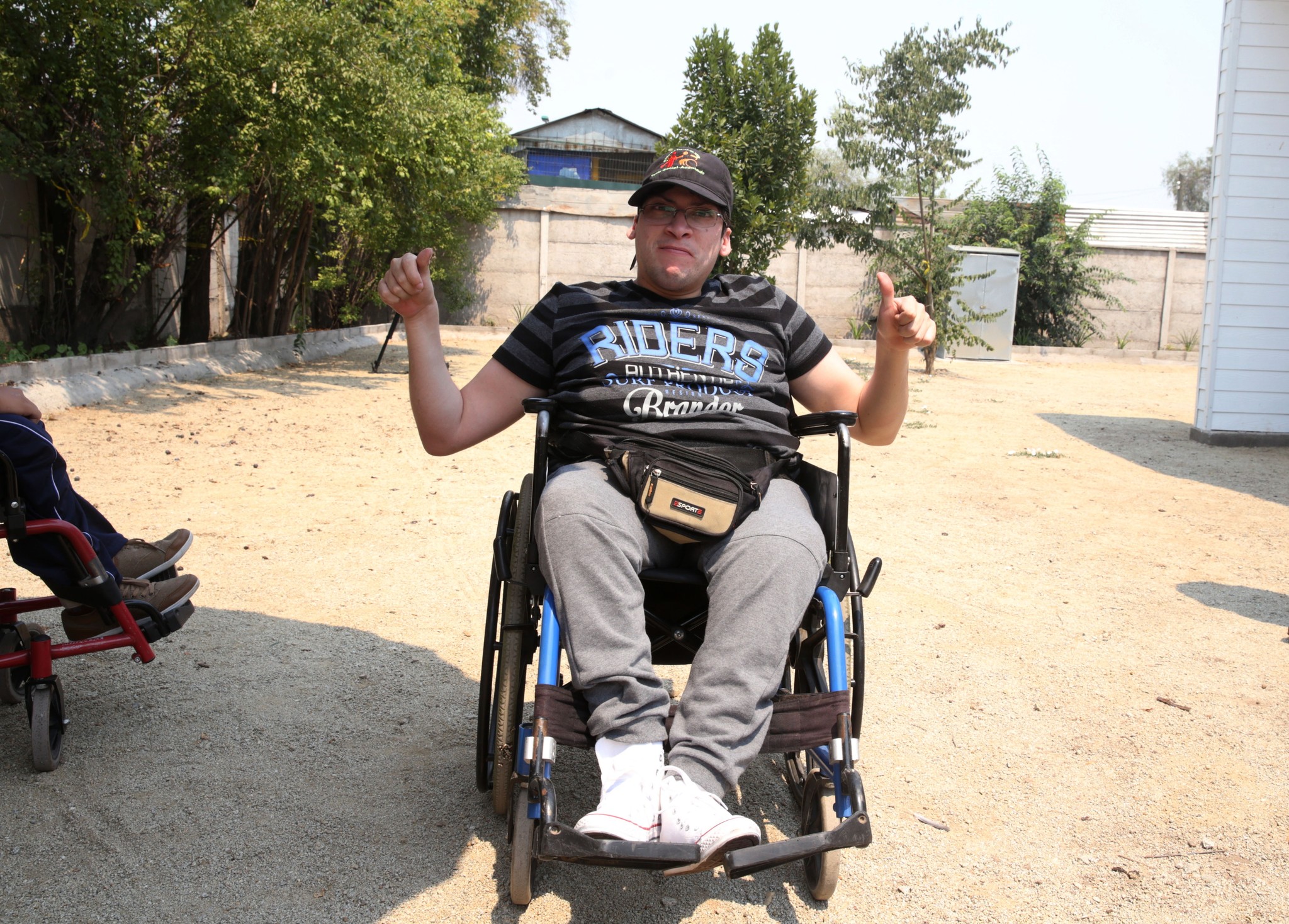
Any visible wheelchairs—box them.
[1,449,195,770]
[476,395,881,906]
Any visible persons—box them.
[1,377,203,641]
[378,147,937,862]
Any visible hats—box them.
[628,147,733,219]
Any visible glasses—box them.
[638,203,729,228]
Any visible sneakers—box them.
[659,765,761,876]
[61,574,200,642]
[574,737,664,841]
[60,529,193,609]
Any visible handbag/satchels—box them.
[605,436,790,544]
